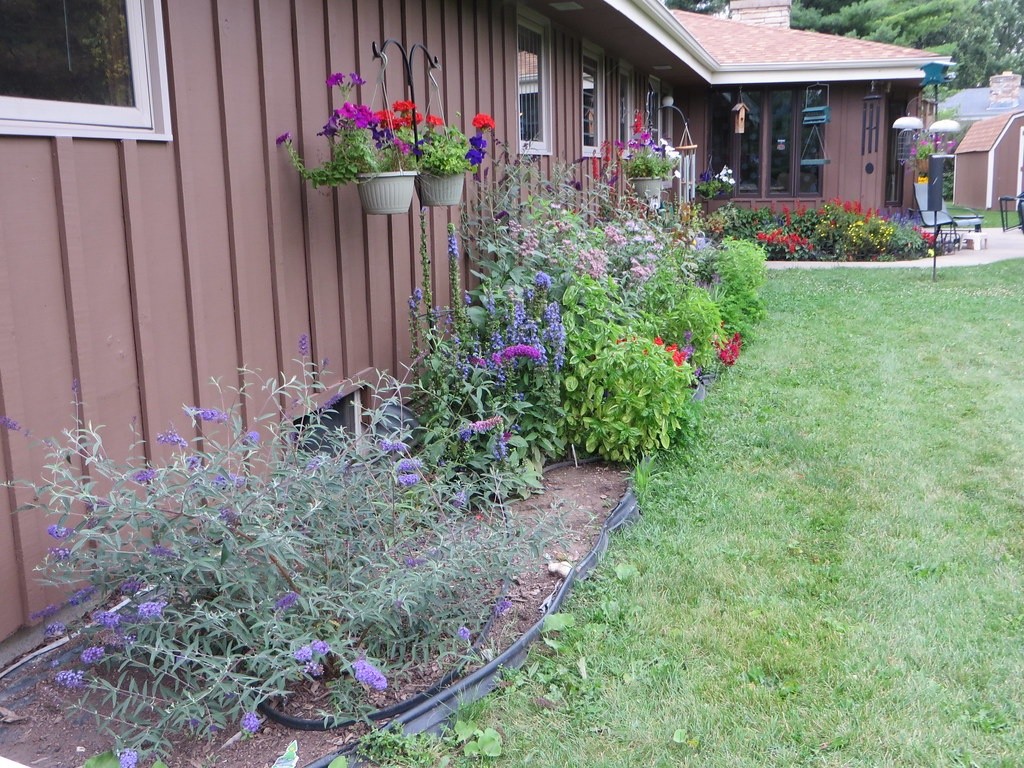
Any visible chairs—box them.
[908,182,985,251]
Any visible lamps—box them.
[662,95,674,106]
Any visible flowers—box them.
[413,111,496,179]
[275,71,423,190]
[900,128,957,166]
[658,137,682,180]
[614,111,666,179]
[695,164,737,200]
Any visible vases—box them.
[355,171,420,215]
[712,185,733,200]
[660,169,673,189]
[916,157,929,173]
[634,176,661,199]
[414,172,465,206]
